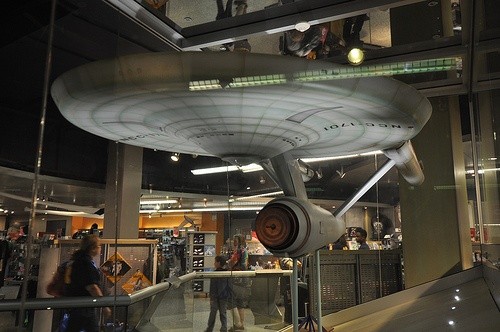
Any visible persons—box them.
[46,250,73,332]
[89,229,94,234]
[204,255,228,332]
[91,223,98,229]
[355,227,370,250]
[223,233,249,331]
[70,234,111,332]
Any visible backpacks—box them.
[45,255,91,296]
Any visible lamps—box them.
[171,153,179,162]
[347,45,365,67]
[336,169,346,178]
[295,22,310,32]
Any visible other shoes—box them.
[239,326,245,330]
[228,326,241,331]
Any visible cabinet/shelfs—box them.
[0,231,158,332]
[139,227,218,297]
